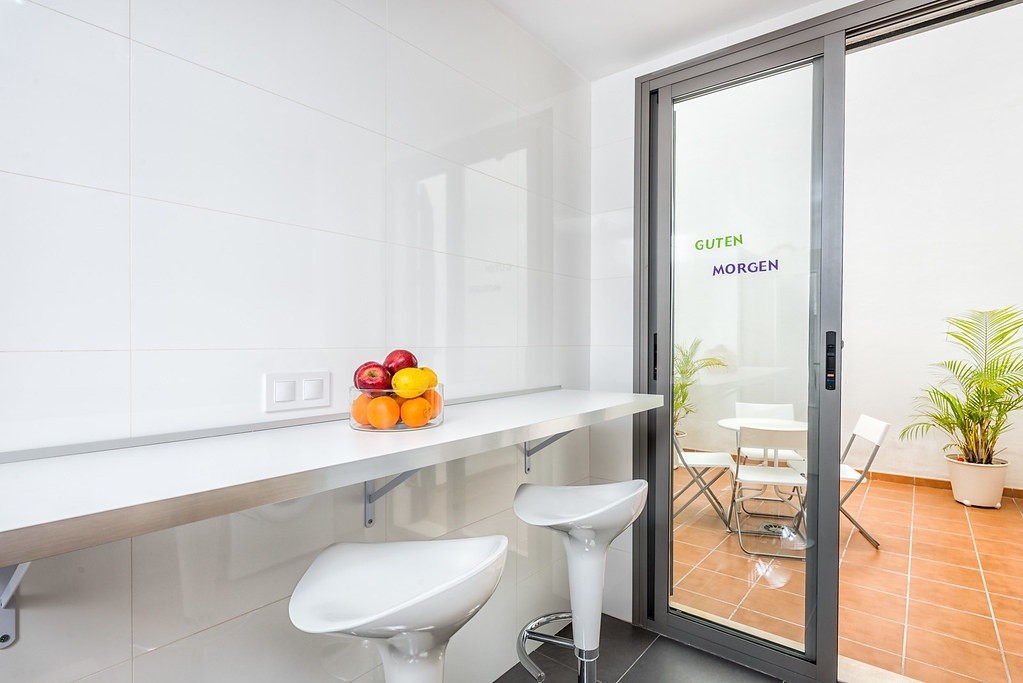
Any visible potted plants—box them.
[674,337,729,470]
[900,302,1023,508]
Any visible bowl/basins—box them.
[348,383,445,433]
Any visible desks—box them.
[717,417,807,536]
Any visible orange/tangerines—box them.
[351,389,442,429]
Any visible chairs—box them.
[729,428,807,560]
[735,402,803,517]
[673,434,735,533]
[787,415,892,550]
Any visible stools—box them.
[288,535,510,683]
[514,478,649,683]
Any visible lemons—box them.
[417,367,437,389]
[391,367,429,399]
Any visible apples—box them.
[353,361,393,398]
[382,349,418,374]
